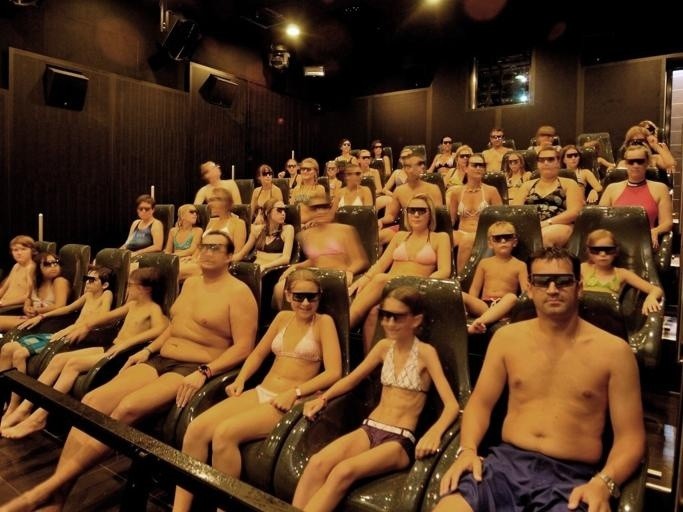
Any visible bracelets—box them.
[143,345,153,355]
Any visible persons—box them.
[585,141,614,182]
[233,198,295,272]
[513,146,583,250]
[482,128,512,173]
[428,136,456,177]
[501,151,531,206]
[194,161,242,206]
[250,165,283,222]
[639,121,669,152]
[0,265,113,418]
[165,204,203,265]
[599,145,672,248]
[617,126,674,170]
[279,159,298,179]
[559,145,602,204]
[0,267,166,438]
[271,193,368,310]
[379,156,443,256]
[581,229,663,316]
[444,145,474,191]
[304,163,373,229]
[349,193,451,354]
[357,149,382,195]
[0,235,35,306]
[289,158,326,204]
[293,285,459,511]
[463,221,530,335]
[325,160,342,197]
[369,140,391,188]
[0,230,258,511]
[118,195,164,272]
[333,139,359,166]
[177,188,247,296]
[434,248,646,512]
[0,252,69,332]
[445,154,502,277]
[375,148,414,211]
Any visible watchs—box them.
[546,217,553,225]
[37,313,44,319]
[597,473,622,497]
[198,363,214,378]
[320,394,330,404]
[294,383,301,397]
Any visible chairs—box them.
[10,248,131,380]
[453,205,545,338]
[394,205,455,282]
[108,262,258,447]
[189,204,210,231]
[331,206,380,283]
[599,165,675,273]
[36,252,181,400]
[273,276,472,511]
[152,205,175,252]
[419,292,651,512]
[559,205,666,369]
[614,129,666,168]
[259,203,301,319]
[0,239,55,315]
[143,131,614,202]
[222,203,252,254]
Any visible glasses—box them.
[127,282,138,287]
[566,153,580,158]
[43,259,60,268]
[327,167,336,171]
[377,308,411,322]
[406,207,427,215]
[471,162,487,169]
[630,138,648,146]
[361,156,371,159]
[83,276,100,284]
[532,273,577,288]
[460,154,472,158]
[402,154,412,159]
[287,165,297,168]
[309,204,330,211]
[138,206,152,211]
[262,171,273,177]
[492,233,513,243]
[491,135,503,139]
[508,159,519,165]
[189,209,198,214]
[444,141,454,145]
[540,133,555,138]
[417,161,427,166]
[588,246,618,255]
[276,207,290,213]
[292,292,321,303]
[537,156,555,163]
[647,127,654,131]
[301,167,315,172]
[355,171,362,177]
[626,158,646,166]
[208,197,221,202]
[198,243,226,252]
[343,143,351,147]
[374,146,382,149]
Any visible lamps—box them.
[286,22,301,40]
